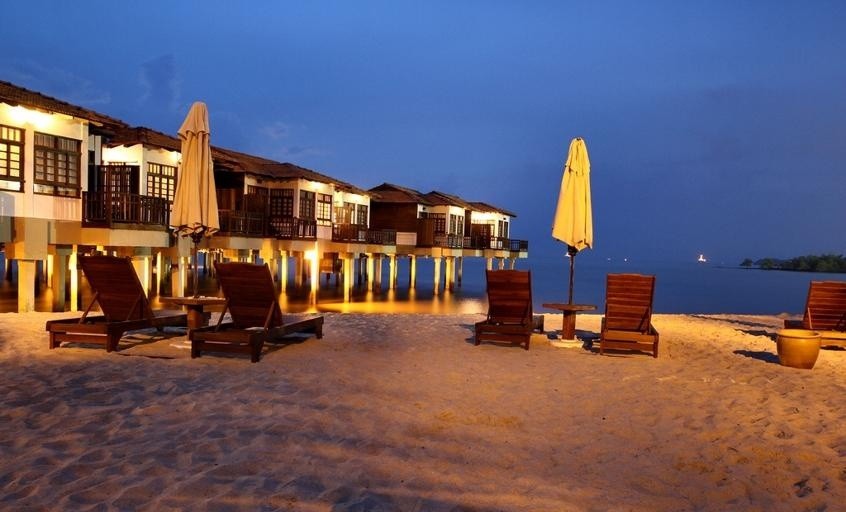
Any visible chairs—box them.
[601,274,659,357]
[784,281,846,351]
[46,254,211,352]
[475,269,544,350]
[190,260,324,363]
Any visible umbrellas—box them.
[170,98,219,294]
[551,137,598,306]
